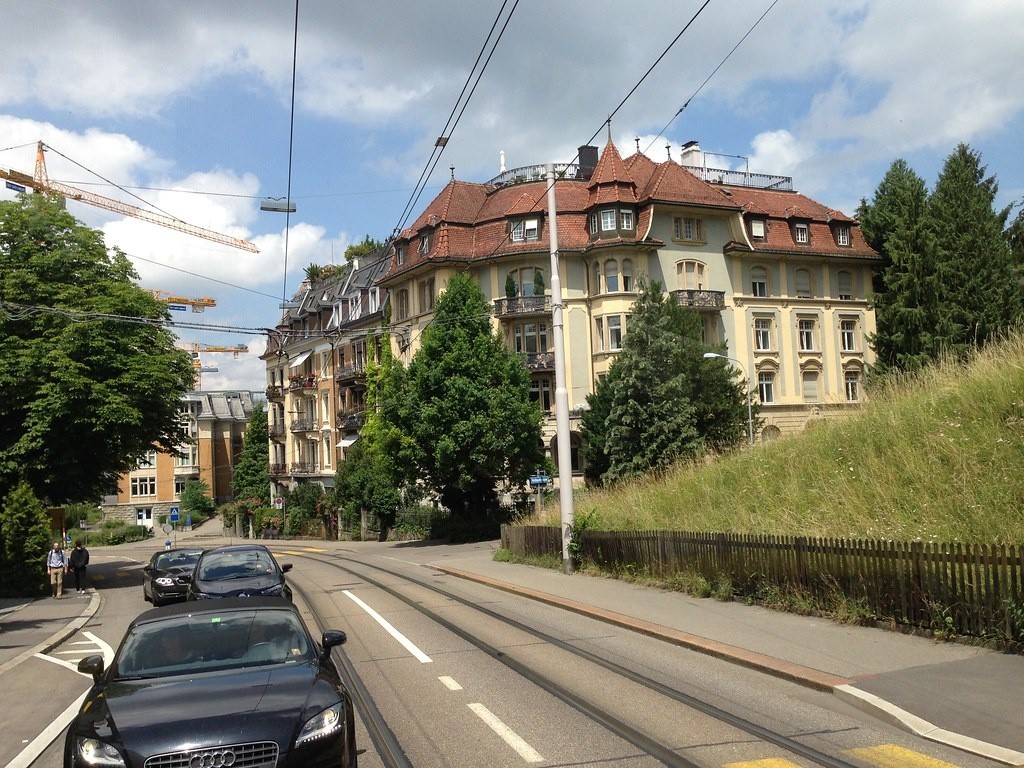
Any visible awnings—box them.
[289,348,315,369]
[335,434,359,448]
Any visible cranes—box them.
[0,141,262,254]
[141,288,217,314]
[178,342,248,393]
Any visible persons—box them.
[47,542,68,599]
[68,540,89,594]
[142,614,297,671]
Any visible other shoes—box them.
[81,590,86,595]
[75,590,81,593]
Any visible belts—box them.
[51,565,63,569]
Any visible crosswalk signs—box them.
[169,507,179,516]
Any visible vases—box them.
[304,380,312,387]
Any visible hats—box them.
[75,541,82,547]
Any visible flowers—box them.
[287,372,316,382]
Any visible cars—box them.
[178,544,293,603]
[63,596,358,768]
[142,546,206,608]
